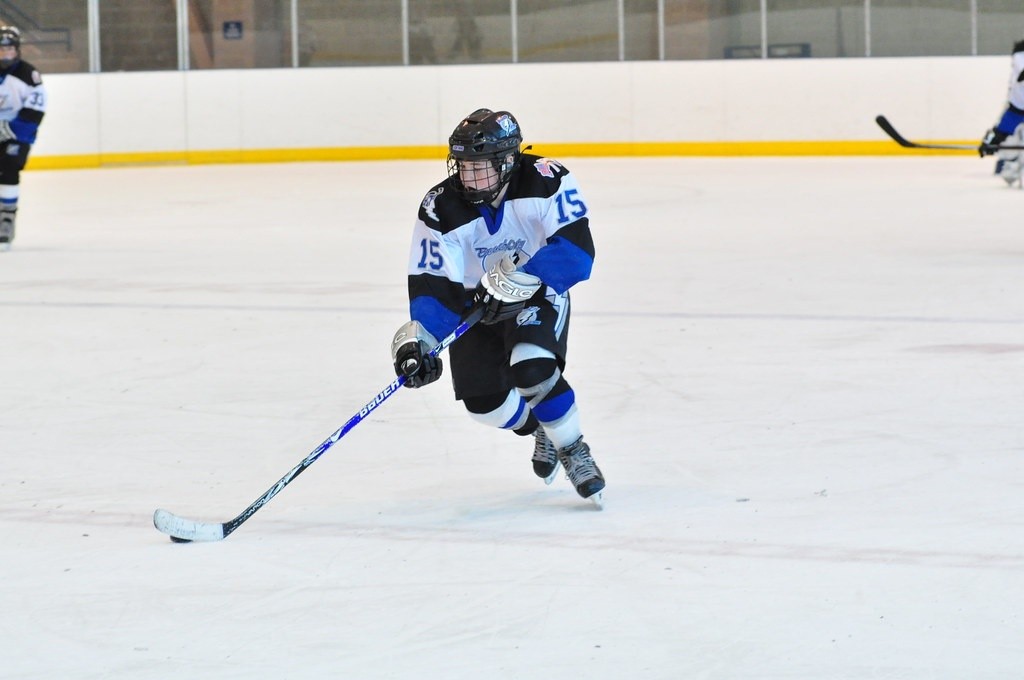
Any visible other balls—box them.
[169,535,192,542]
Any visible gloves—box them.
[391,320,442,389]
[979,127,1007,158]
[0,120,16,143]
[471,254,542,326]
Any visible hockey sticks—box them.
[875,114,1024,153]
[152,305,487,543]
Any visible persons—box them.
[979,37,1024,190]
[0,23,47,252]
[389,108,606,507]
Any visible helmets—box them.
[0,26,20,69]
[446,108,532,205]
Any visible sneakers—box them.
[513,405,561,485]
[557,435,606,511]
[0,205,17,250]
